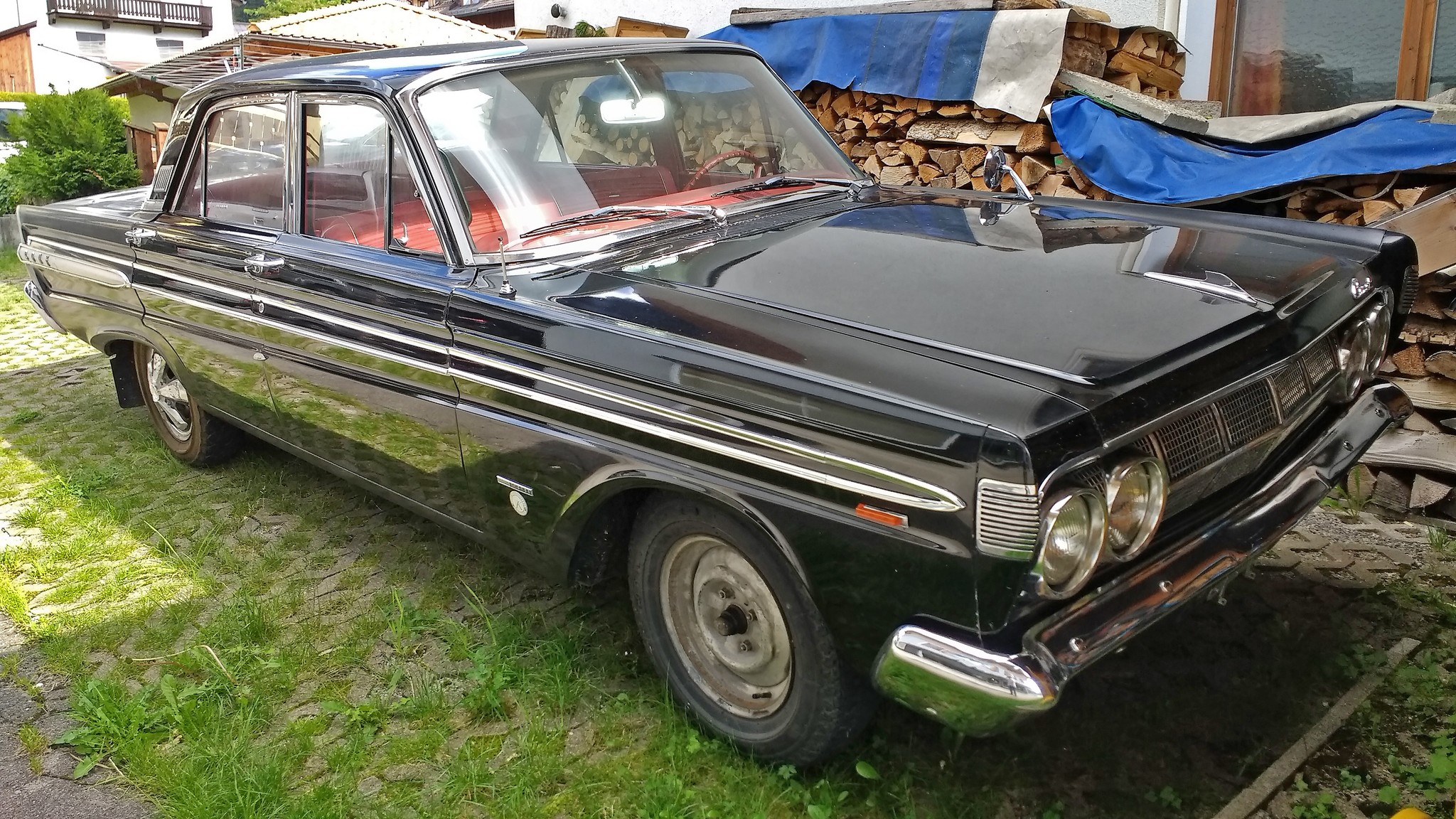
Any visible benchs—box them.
[192,164,678,254]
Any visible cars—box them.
[0,102,33,165]
[13,39,1424,768]
